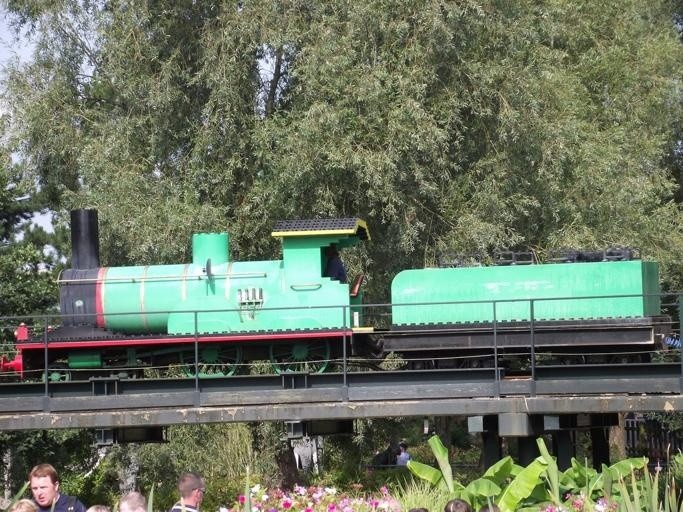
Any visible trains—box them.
[1,209,673,380]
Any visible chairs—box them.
[350,274,364,296]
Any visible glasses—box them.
[192,487,206,493]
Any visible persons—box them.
[323,242,347,282]
[86,502,107,512]
[443,498,472,512]
[406,507,429,512]
[395,443,413,466]
[478,504,500,512]
[6,499,42,512]
[28,462,87,512]
[168,471,206,512]
[117,491,148,512]
